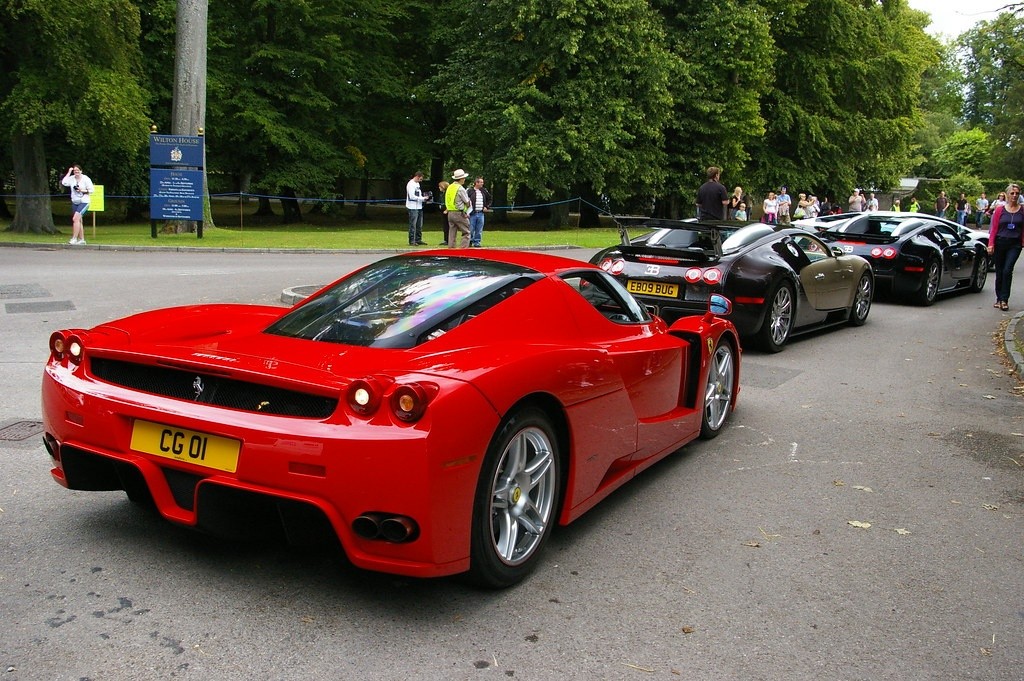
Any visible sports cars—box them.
[580,215,877,354]
[788,211,995,234]
[41,247,741,587]
[812,212,989,307]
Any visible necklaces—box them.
[989,192,1006,233]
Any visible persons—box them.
[986,184,1024,311]
[975,192,989,230]
[730,186,743,219]
[62,165,94,246]
[954,194,971,226]
[890,200,900,211]
[1017,194,1024,205]
[466,177,492,247]
[763,186,820,224]
[934,191,950,218]
[910,198,921,212]
[848,188,878,212]
[438,181,450,245]
[696,166,729,221]
[444,169,471,248]
[736,203,747,221]
[405,171,429,246]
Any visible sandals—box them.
[994,300,1001,308]
[1000,300,1008,310]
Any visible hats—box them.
[452,169,469,180]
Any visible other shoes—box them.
[417,240,428,246]
[440,241,448,245]
[475,244,481,247]
[468,242,472,247]
[410,243,418,246]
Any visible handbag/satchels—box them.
[988,200,997,215]
[793,205,805,219]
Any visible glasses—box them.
[1009,192,1020,195]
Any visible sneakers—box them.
[77,240,87,246]
[70,239,77,246]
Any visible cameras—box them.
[74,185,79,191]
